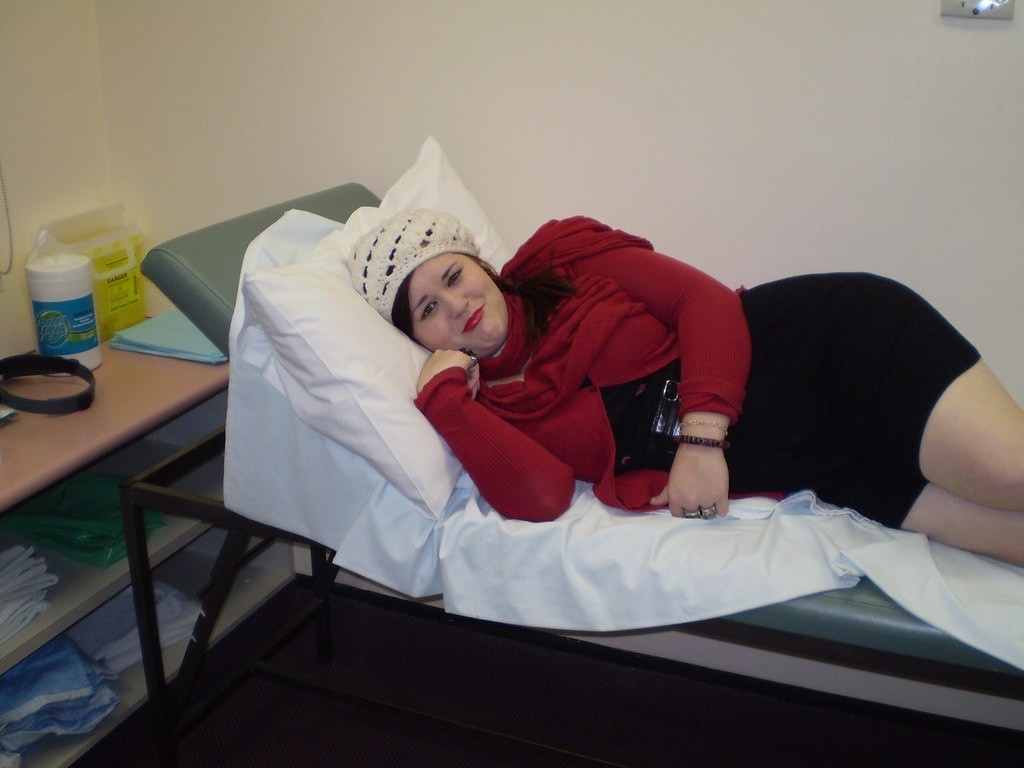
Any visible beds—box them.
[122,182,1024,731]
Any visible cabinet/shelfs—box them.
[0,309,298,768]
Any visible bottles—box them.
[27,252,102,376]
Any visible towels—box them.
[0,470,204,753]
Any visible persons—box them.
[345,208,1024,567]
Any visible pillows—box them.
[243,135,509,524]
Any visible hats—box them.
[352,209,478,326]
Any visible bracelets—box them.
[680,419,728,436]
[675,435,730,449]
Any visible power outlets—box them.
[938,0,1014,20]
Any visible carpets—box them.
[62,570,1024,768]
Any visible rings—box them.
[460,348,473,355]
[701,506,717,519]
[684,509,701,518]
[469,353,477,366]
